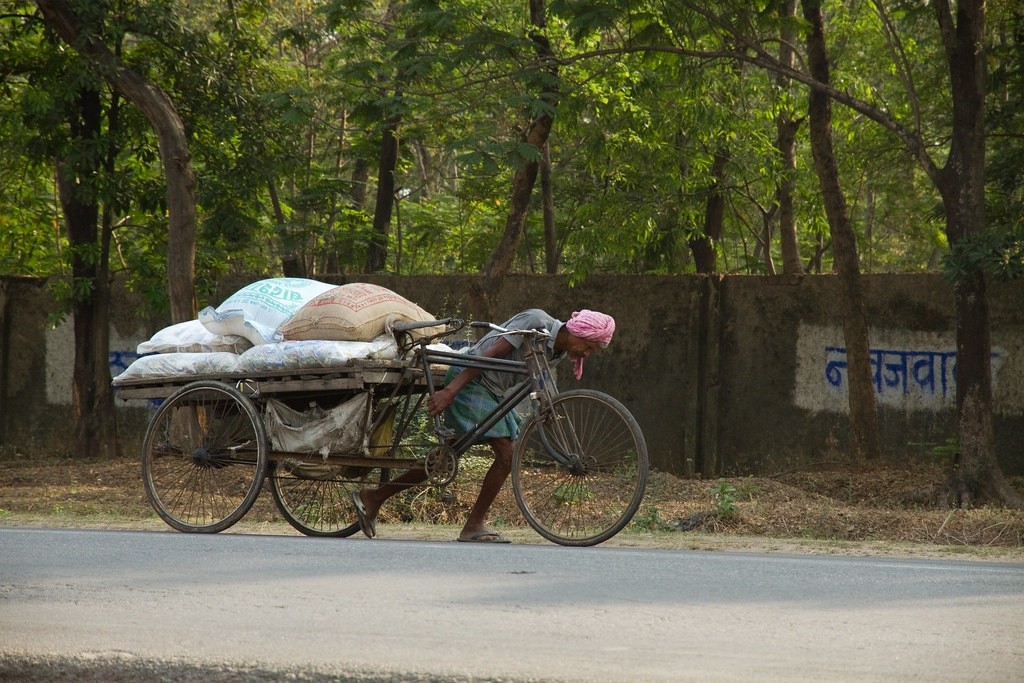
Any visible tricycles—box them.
[112,317,650,547]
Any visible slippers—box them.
[352,489,382,538]
[455,531,511,542]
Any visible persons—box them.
[350,308,616,544]
